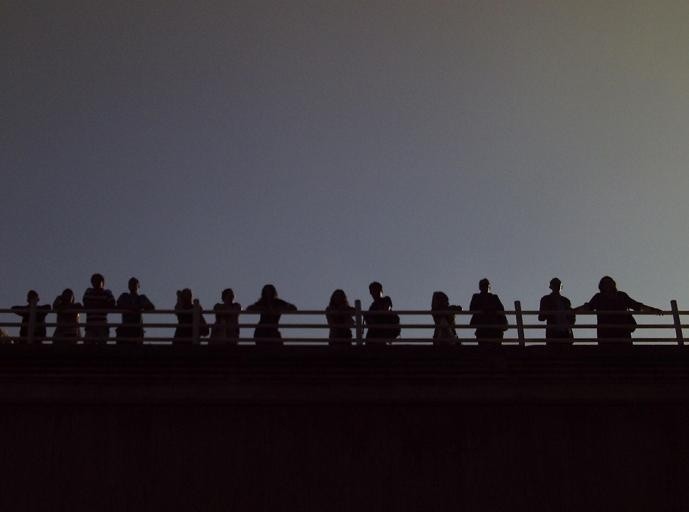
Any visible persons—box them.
[536,277,578,346]
[10,288,53,344]
[114,275,156,344]
[573,275,666,345]
[82,271,117,346]
[245,284,298,346]
[53,286,84,346]
[208,287,243,347]
[169,287,210,346]
[468,277,509,346]
[323,288,358,346]
[361,281,402,345]
[429,289,464,346]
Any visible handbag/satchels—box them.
[200,316,210,337]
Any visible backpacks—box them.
[386,310,402,344]
